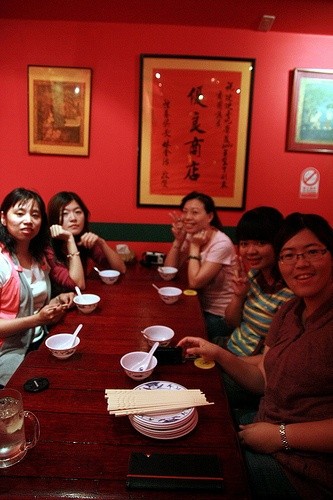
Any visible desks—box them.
[1,260,251,500]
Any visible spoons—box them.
[63,324,83,349]
[130,341,159,372]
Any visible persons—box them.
[212,205,294,413]
[0,187,75,390]
[164,191,239,342]
[176,212,333,500]
[40,191,126,299]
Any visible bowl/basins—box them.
[142,325,175,348]
[157,266,178,280]
[158,287,182,304]
[99,269,121,284]
[45,333,81,360]
[120,351,157,382]
[73,294,101,313]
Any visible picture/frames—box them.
[26,64,92,157]
[285,66,333,155]
[136,53,256,212]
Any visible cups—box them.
[0,387,41,468]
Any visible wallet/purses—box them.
[125,452,225,495]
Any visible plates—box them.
[128,381,199,441]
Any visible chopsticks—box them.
[103,388,215,416]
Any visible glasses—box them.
[277,248,328,264]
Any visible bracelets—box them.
[280,422,291,451]
[188,256,199,260]
[67,251,80,258]
[173,242,179,249]
